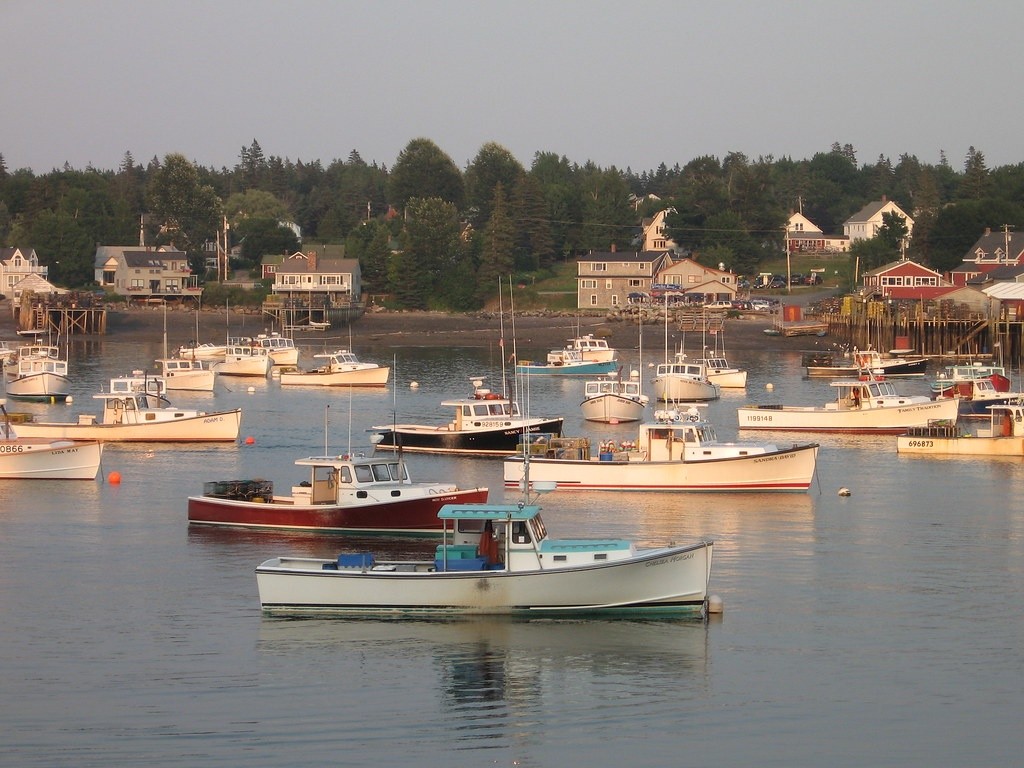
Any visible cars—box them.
[623,255,825,314]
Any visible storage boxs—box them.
[338,553,375,571]
[0,414,26,423]
[204,479,274,504]
[10,412,33,421]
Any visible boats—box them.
[581,304,648,424]
[281,310,392,389]
[501,279,822,495]
[695,311,749,391]
[369,276,562,455]
[735,298,1024,467]
[1,318,71,403]
[180,332,228,358]
[185,344,489,547]
[230,313,300,366]
[9,373,245,445]
[0,396,107,486]
[207,297,275,379]
[653,292,720,405]
[133,295,219,392]
[516,301,623,378]
[254,365,713,618]
